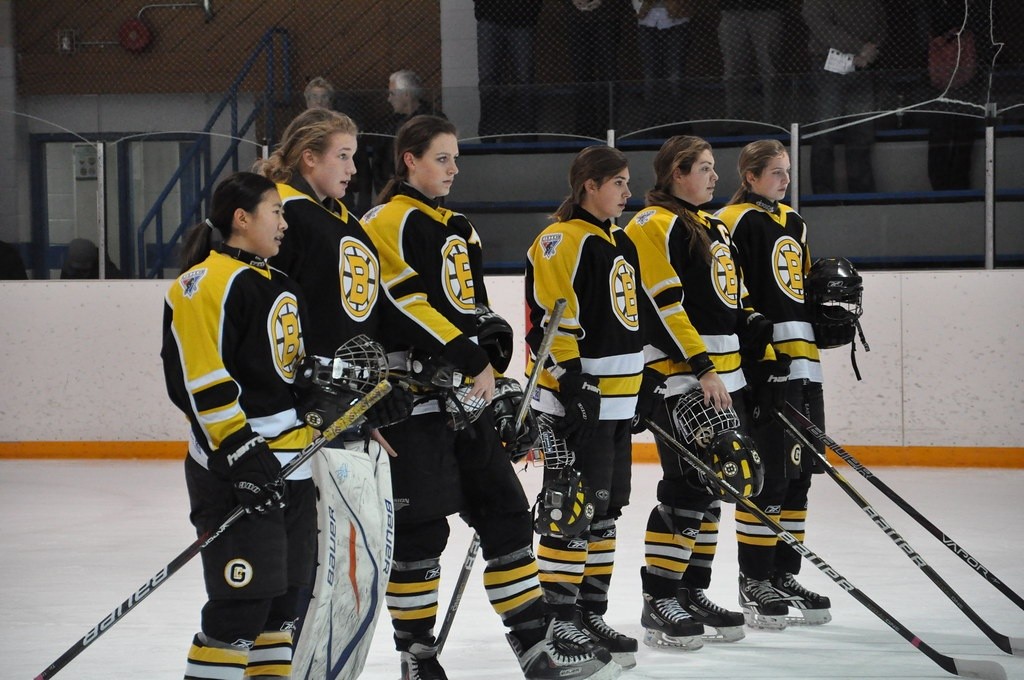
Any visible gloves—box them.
[750,352,794,409]
[206,423,294,523]
[557,372,601,449]
[816,325,858,349]
[628,367,668,434]
[487,377,541,465]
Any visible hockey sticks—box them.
[648,422,1007,680]
[773,410,1024,658]
[314,355,478,440]
[30,377,394,680]
[785,402,1023,611]
[433,297,569,655]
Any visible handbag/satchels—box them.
[927,26,979,92]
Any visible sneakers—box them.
[504,609,622,680]
[737,566,789,631]
[770,571,832,627]
[675,565,745,643]
[392,632,448,680]
[552,599,638,670]
[639,565,705,651]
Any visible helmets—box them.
[673,385,766,504]
[805,255,864,328]
[293,334,392,435]
[535,465,597,542]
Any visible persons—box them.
[475,0,988,206]
[357,69,440,203]
[624,135,777,651]
[161,173,397,680]
[712,140,832,631]
[304,76,338,110]
[532,145,650,680]
[358,115,624,680]
[60,238,126,279]
[251,108,414,680]
[0,240,30,280]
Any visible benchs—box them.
[447,111,1024,273]
